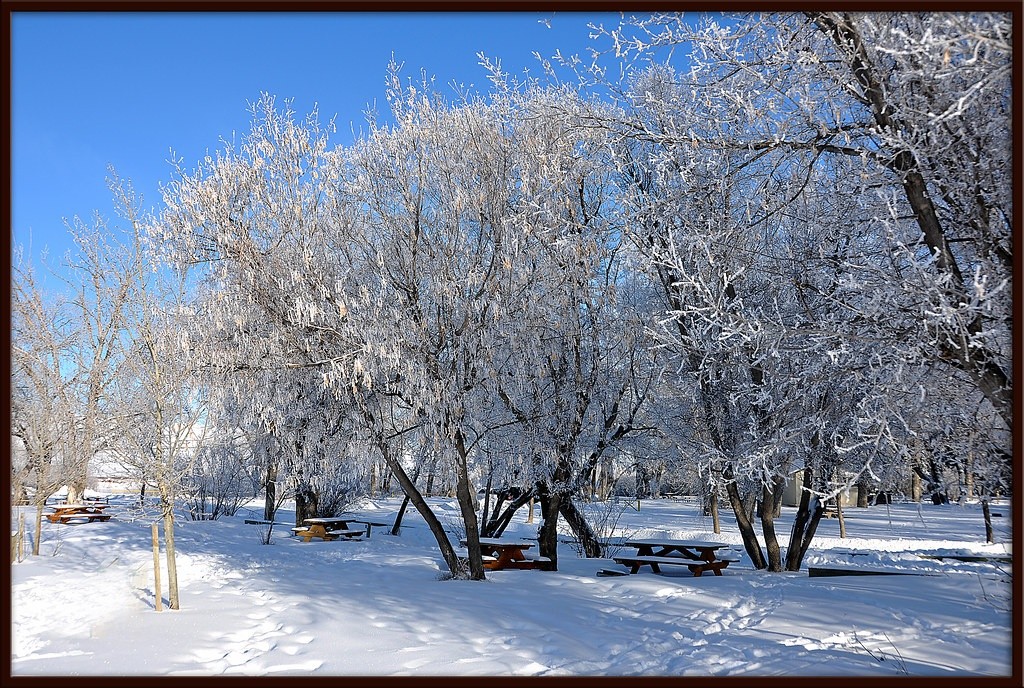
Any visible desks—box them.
[47,505,110,524]
[626,539,729,577]
[460,538,535,571]
[302,517,356,542]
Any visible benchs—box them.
[613,556,709,573]
[61,514,111,524]
[492,552,551,567]
[456,553,497,568]
[664,553,740,569]
[41,513,56,518]
[325,530,366,539]
[291,526,332,537]
